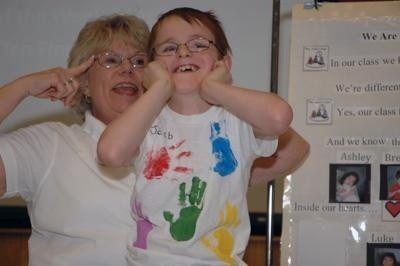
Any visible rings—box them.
[64,79,72,86]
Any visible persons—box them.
[381,252,400,265]
[337,171,361,202]
[97,6,293,266]
[0,13,311,266]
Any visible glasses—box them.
[93,53,147,69]
[151,37,216,56]
